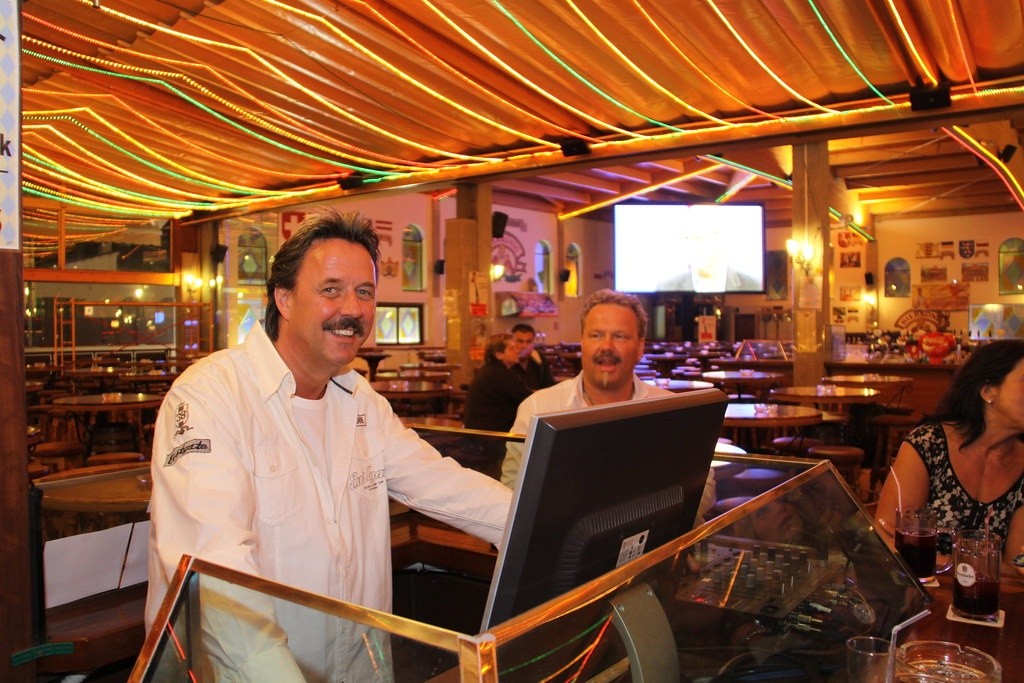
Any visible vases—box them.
[866,346,886,363]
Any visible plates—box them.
[136,474,151,483]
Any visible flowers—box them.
[866,325,890,352]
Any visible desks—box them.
[377,370,450,382]
[371,380,453,416]
[66,367,128,393]
[549,343,913,454]
[119,370,181,393]
[901,591,1024,683]
[26,380,45,394]
[119,360,187,370]
[65,358,119,367]
[32,463,152,539]
[53,393,161,467]
[26,365,63,389]
[357,352,392,381]
[399,362,463,372]
[403,415,468,449]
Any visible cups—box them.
[893,505,956,584]
[952,530,1002,621]
[846,637,895,683]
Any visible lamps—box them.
[436,258,445,275]
[337,172,362,191]
[491,210,509,239]
[559,137,591,156]
[1000,143,1017,164]
[865,271,875,283]
[207,241,228,263]
[184,274,201,303]
[908,83,952,111]
[560,269,571,282]
[785,236,813,275]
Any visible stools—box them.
[29,441,144,478]
[727,392,913,507]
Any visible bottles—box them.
[541,333,546,344]
[953,329,993,345]
[536,332,541,344]
[908,335,913,344]
[866,330,870,340]
[870,334,874,340]
[847,336,861,345]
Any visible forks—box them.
[936,559,953,574]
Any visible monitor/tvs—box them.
[478,387,730,683]
[614,202,767,295]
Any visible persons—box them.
[874,339,1024,574]
[501,287,716,573]
[145,203,514,683]
[464,323,561,432]
